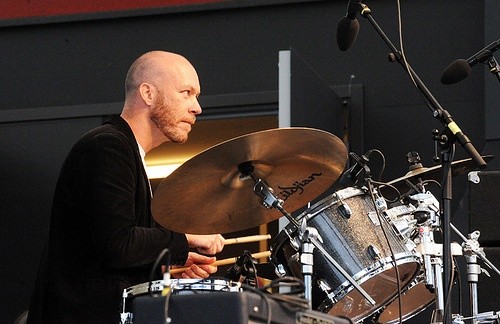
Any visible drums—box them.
[368,274,435,324]
[268,184,423,321]
[119,278,247,324]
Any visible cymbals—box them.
[149,126,351,235]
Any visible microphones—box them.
[226,253,248,280]
[340,150,372,186]
[338,0,363,52]
[440,39,500,85]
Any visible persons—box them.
[26,51,225,324]
